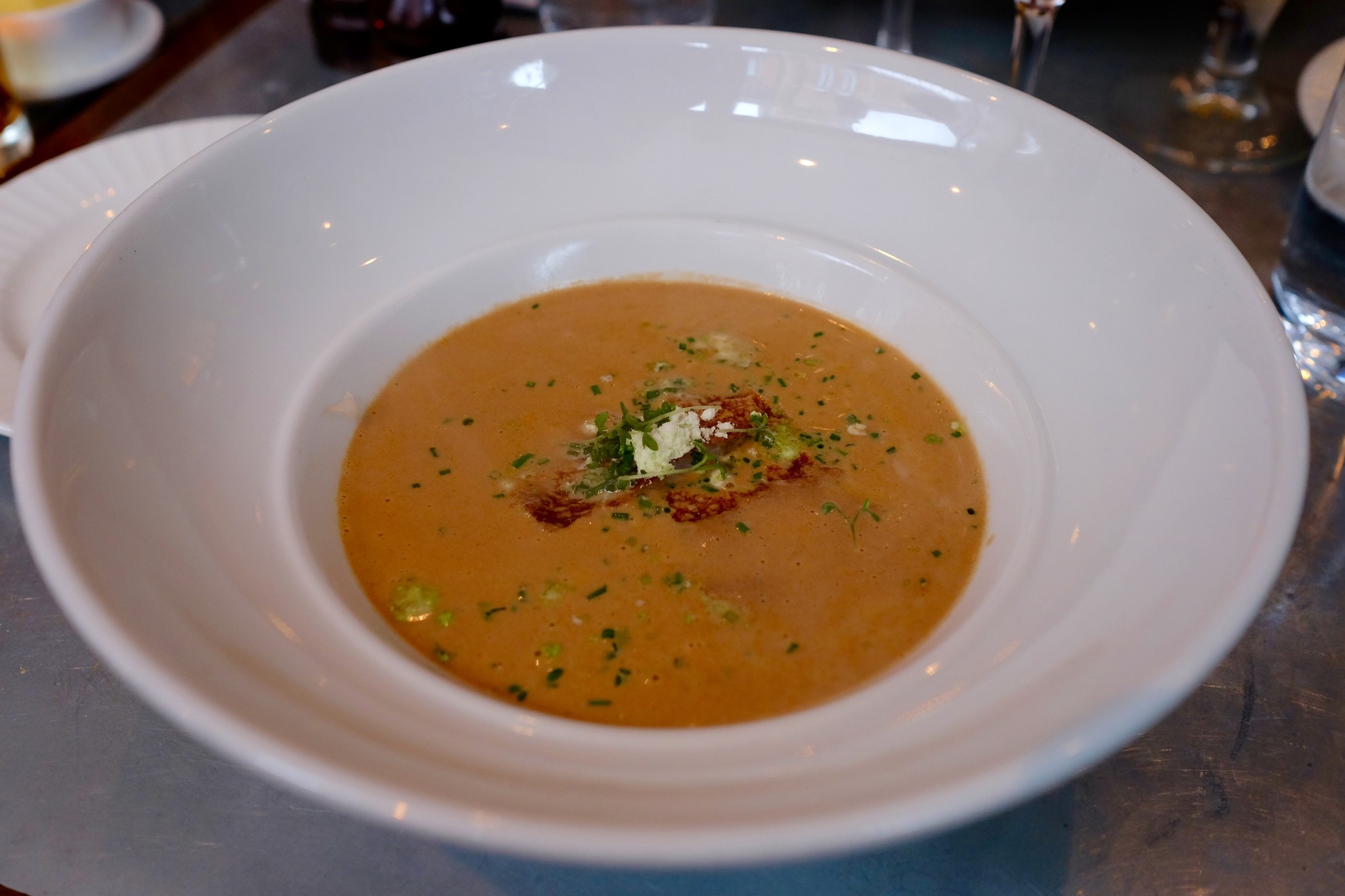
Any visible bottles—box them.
[1272,25,1345,417]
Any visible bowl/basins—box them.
[12,13,1310,886]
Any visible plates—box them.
[2,0,164,95]
[3,115,269,438]
